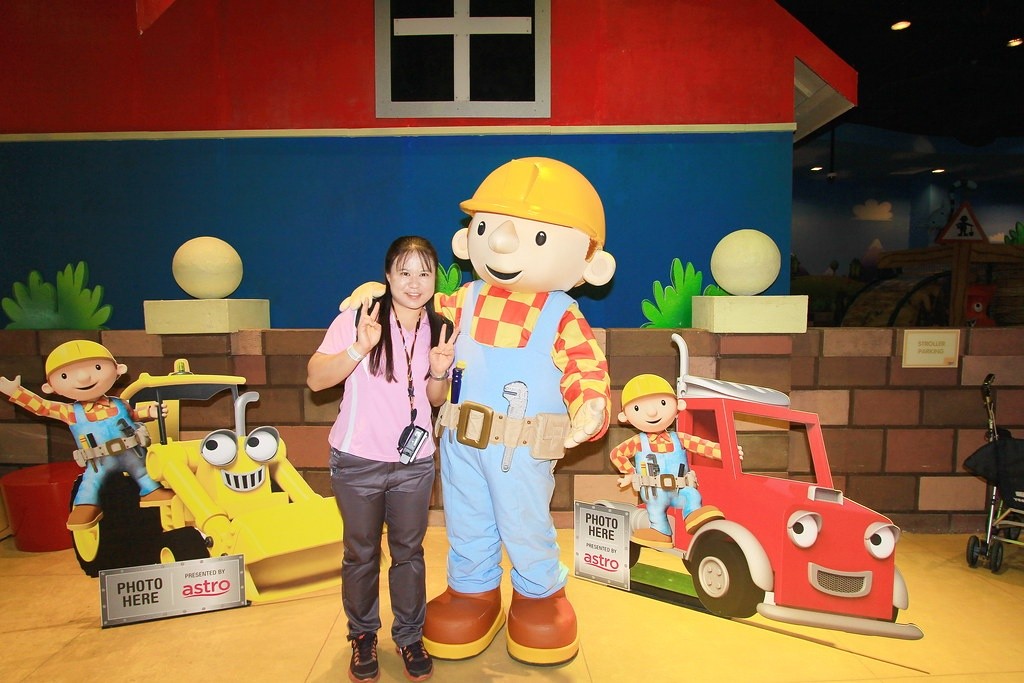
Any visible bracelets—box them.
[346,342,367,363]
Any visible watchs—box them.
[429,369,450,381]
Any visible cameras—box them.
[398,426,428,464]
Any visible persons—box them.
[305,236,462,683]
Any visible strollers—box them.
[960,374,1024,572]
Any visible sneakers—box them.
[396,638,434,682]
[348,631,380,683]
